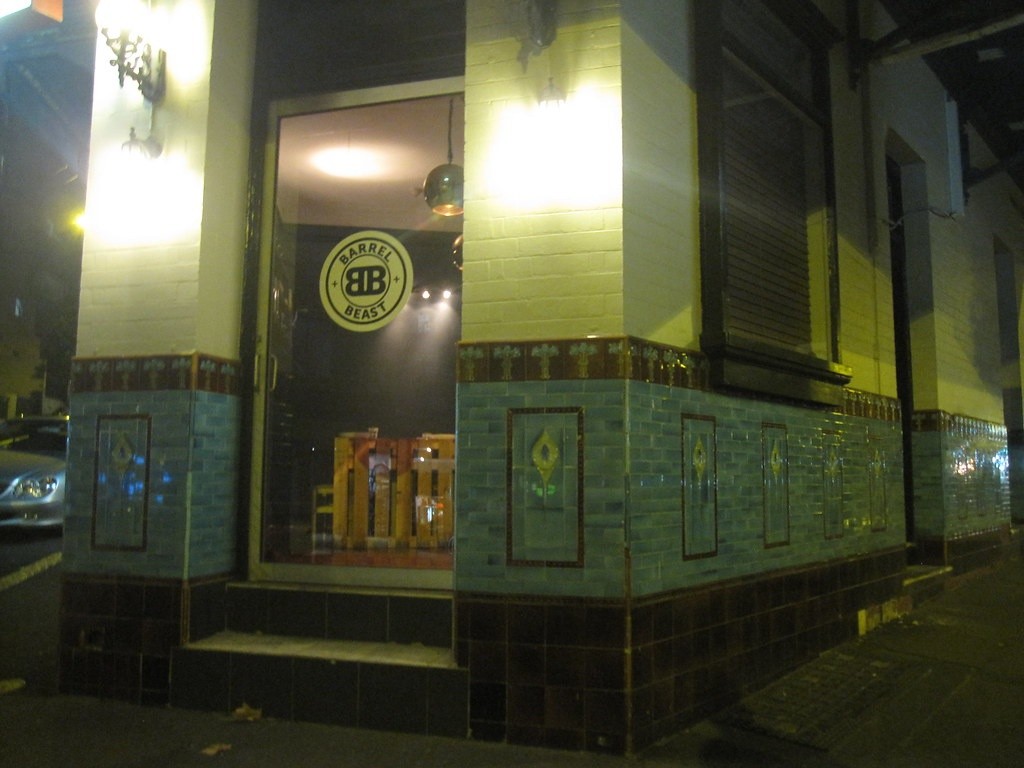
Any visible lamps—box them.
[424,95,464,216]
[95,0,168,159]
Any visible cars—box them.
[1,412,74,537]
